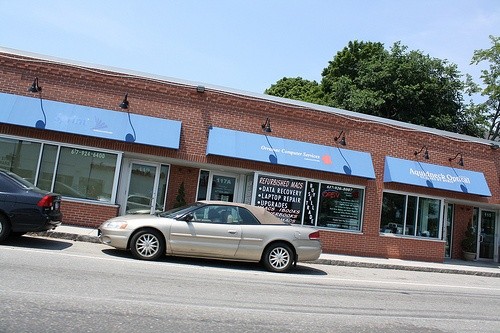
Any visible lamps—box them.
[414,145,431,160]
[119,94,129,109]
[261,118,272,133]
[29,77,41,93]
[334,130,347,146]
[449,153,464,167]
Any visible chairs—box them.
[222,214,233,224]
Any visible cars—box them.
[0,167,63,244]
[96,194,163,214]
[22,177,96,200]
[97,200,323,273]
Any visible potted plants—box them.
[460,218,476,261]
